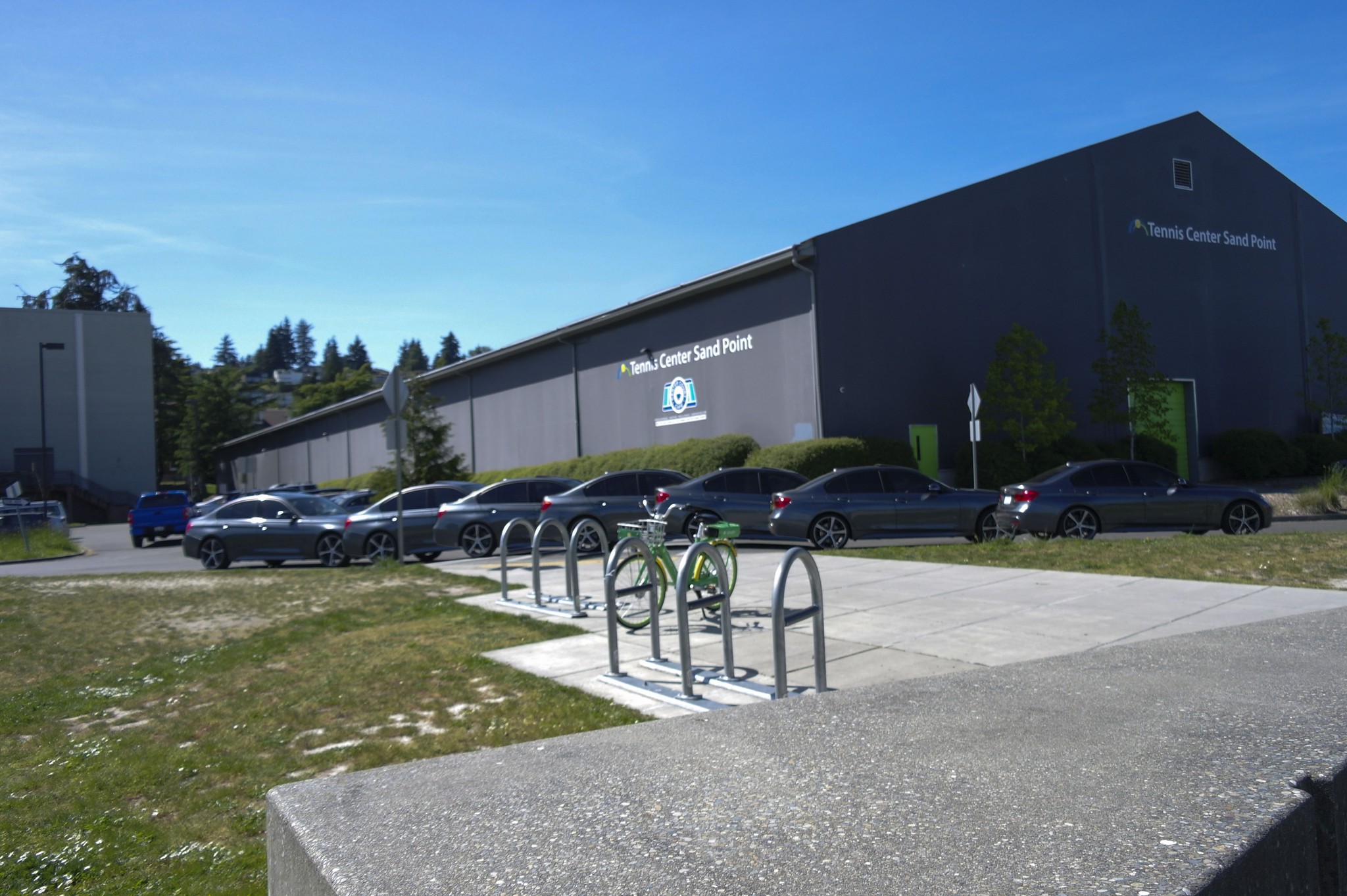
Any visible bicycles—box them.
[614,495,741,629]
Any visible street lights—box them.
[38,341,65,528]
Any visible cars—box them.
[432,475,588,558]
[182,492,354,570]
[535,468,696,554]
[768,463,1001,545]
[996,459,1275,543]
[342,481,488,565]
[192,481,378,518]
[127,490,196,549]
[653,466,811,547]
